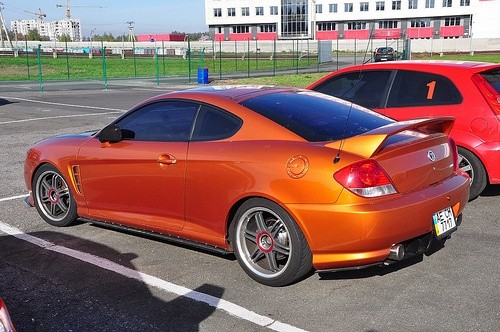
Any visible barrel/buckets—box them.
[198,68,208,84]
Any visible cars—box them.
[374,47,397,62]
[305,60,500,201]
[24,84,471,286]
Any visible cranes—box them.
[56,0,102,19]
[25,7,47,20]
